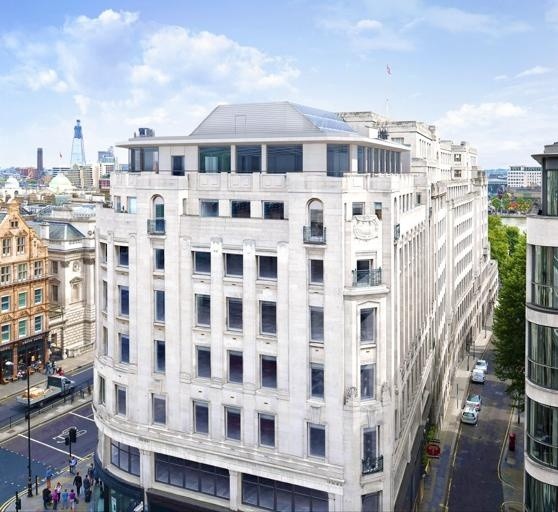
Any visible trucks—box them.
[16,375,76,407]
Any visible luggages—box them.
[85,487,91,502]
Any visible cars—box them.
[470,368,485,384]
[474,360,487,373]
[463,393,481,411]
[460,405,478,424]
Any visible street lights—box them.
[4,360,33,498]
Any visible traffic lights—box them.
[64,437,69,445]
[15,500,20,509]
[69,428,76,443]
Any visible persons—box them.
[41,456,96,512]
[15,357,66,380]
